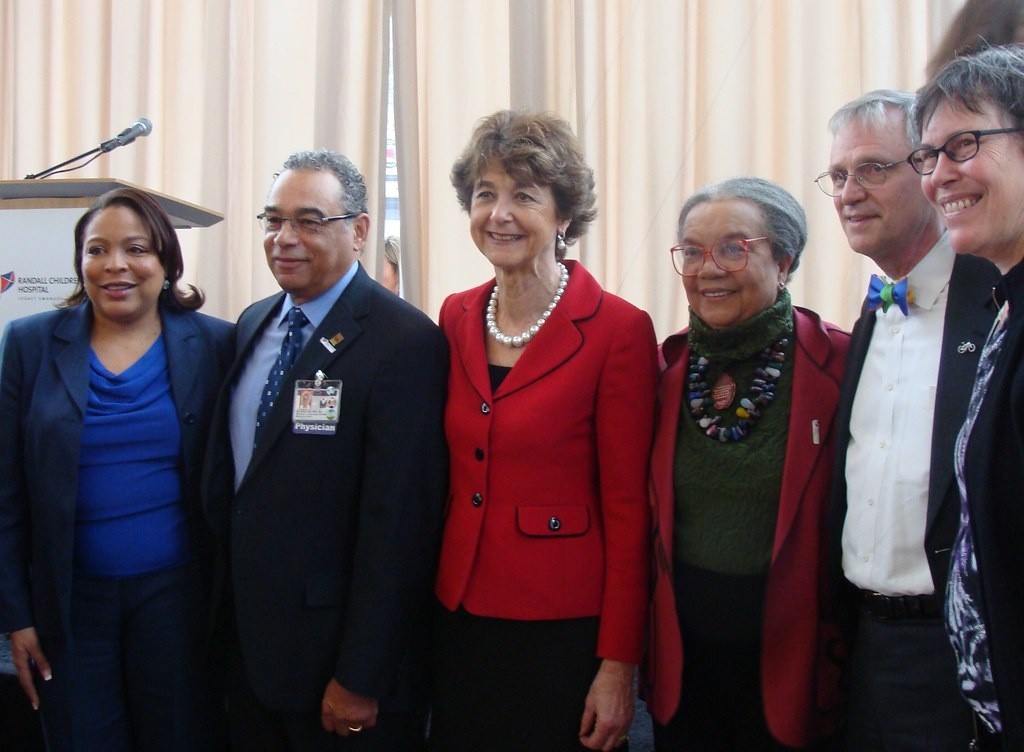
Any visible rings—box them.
[349,725,363,732]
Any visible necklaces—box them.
[686,334,786,442]
[485,262,570,348]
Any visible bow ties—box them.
[868,273,910,318]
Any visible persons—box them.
[635,178,853,752]
[0,186,239,751]
[200,151,451,752]
[434,108,661,752]
[814,88,999,752]
[905,43,1023,752]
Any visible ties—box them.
[254,306,308,456]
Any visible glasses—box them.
[669,236,769,279]
[907,128,1020,175]
[814,159,908,197]
[257,212,356,232]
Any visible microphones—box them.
[103,118,152,153]
